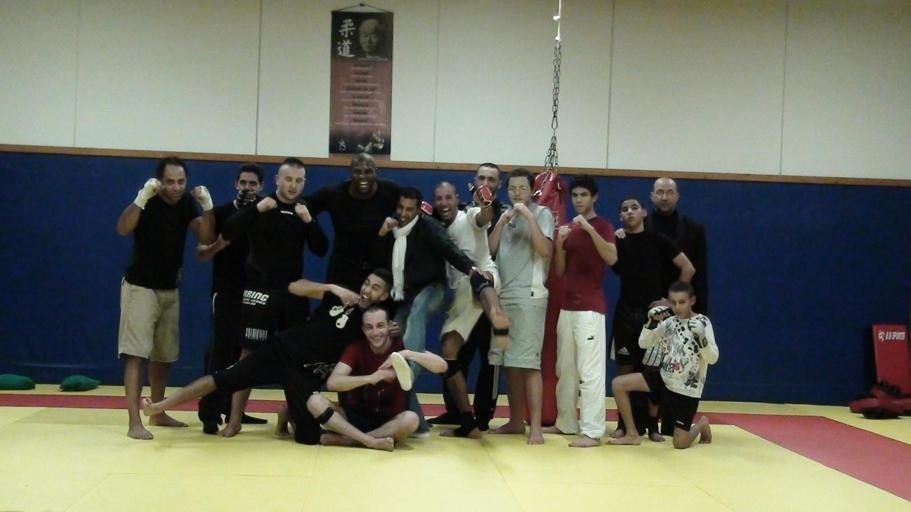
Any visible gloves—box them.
[687,318,708,348]
[191,185,214,212]
[645,306,670,330]
[473,185,495,209]
[237,187,259,208]
[420,201,433,216]
[133,178,164,211]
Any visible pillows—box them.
[60,374,101,392]
[1,374,36,390]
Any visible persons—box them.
[138,268,397,446]
[426,163,513,431]
[636,176,708,437]
[608,199,697,445]
[300,154,412,305]
[420,181,511,439]
[555,177,619,447]
[197,165,270,433]
[225,159,330,436]
[610,280,720,447]
[487,169,554,445]
[357,18,391,61]
[116,153,219,439]
[375,185,485,438]
[307,305,451,447]
